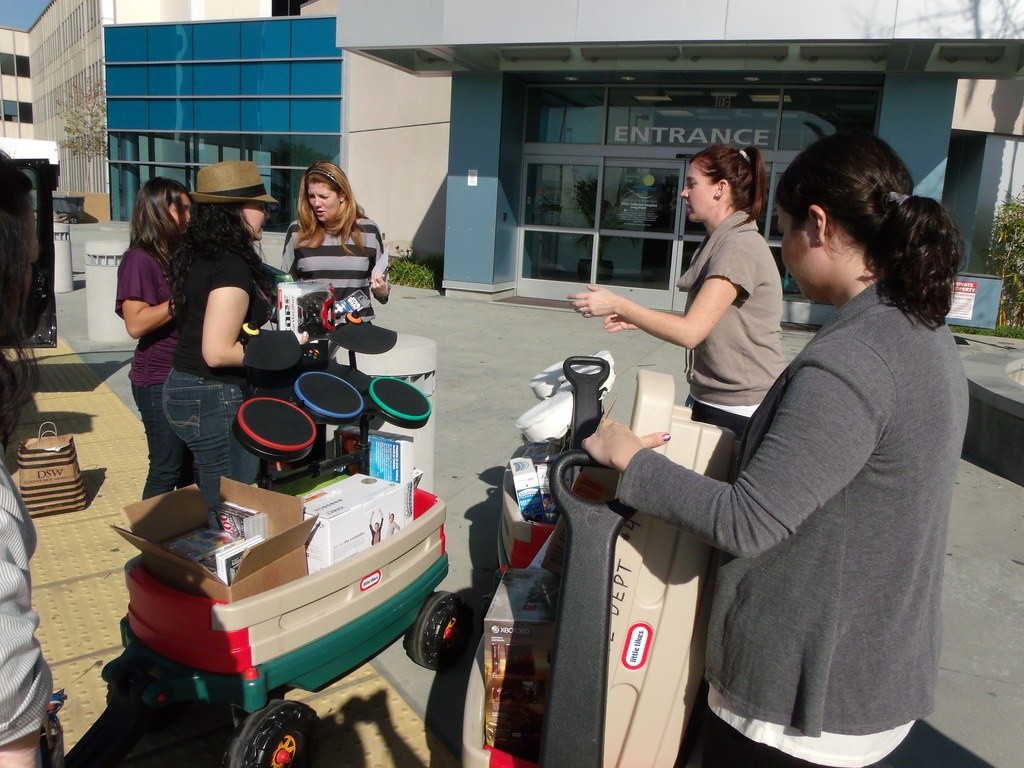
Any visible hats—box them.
[189,160,279,203]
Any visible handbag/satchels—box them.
[17,422,86,518]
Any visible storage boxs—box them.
[276,280,336,341]
[484,567,561,752]
[510,456,547,521]
[500,444,556,568]
[333,424,415,483]
[109,476,319,604]
[298,466,423,573]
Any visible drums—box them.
[232,371,431,460]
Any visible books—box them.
[156,501,267,587]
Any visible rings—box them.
[581,307,584,313]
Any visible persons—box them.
[386,513,400,537]
[369,509,384,545]
[162,162,307,515]
[581,130,969,768]
[0,149,53,768]
[115,177,199,501]
[281,162,391,328]
[567,144,783,440]
[543,493,551,511]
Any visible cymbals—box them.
[245,328,301,370]
[328,321,398,355]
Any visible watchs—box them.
[374,289,390,301]
